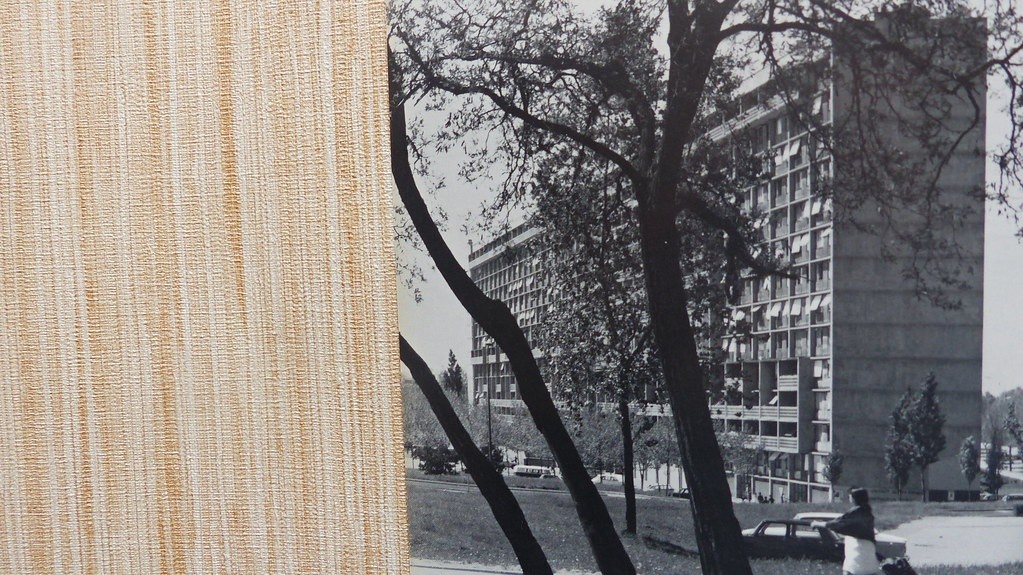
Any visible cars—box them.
[672,487,690,499]
[593,475,622,487]
[741,519,884,564]
[540,474,559,481]
[791,512,907,560]
[647,483,673,496]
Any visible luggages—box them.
[876,551,916,575]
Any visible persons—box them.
[810,487,880,575]
[757,492,775,504]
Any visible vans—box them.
[514,464,551,478]
